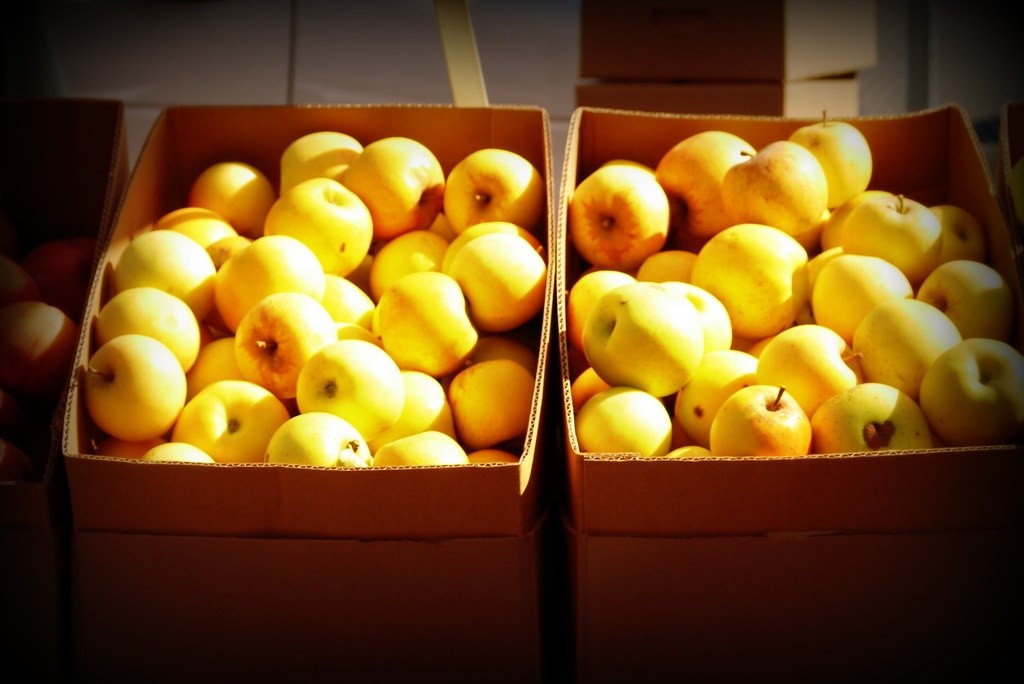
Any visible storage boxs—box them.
[0,0,1024,684]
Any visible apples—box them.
[84,130,546,466]
[566,107,1020,458]
[0,229,97,480]
